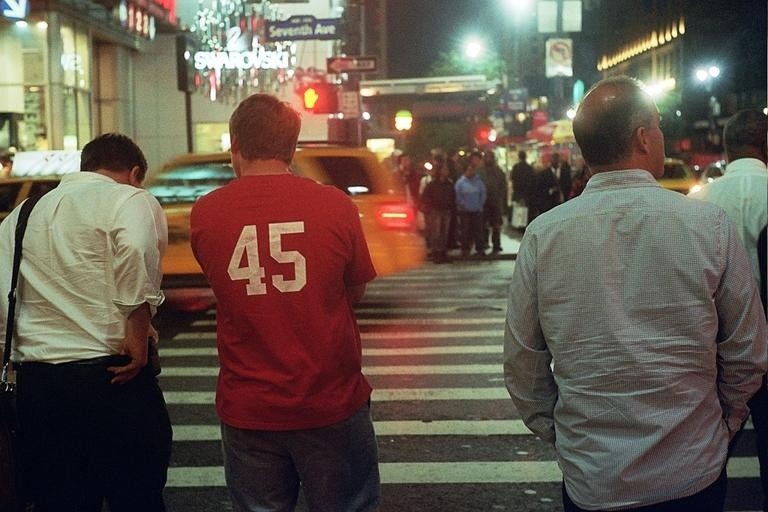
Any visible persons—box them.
[0,130,175,512]
[394,145,590,268]
[684,105,768,509]
[502,74,768,509]
[189,93,382,510]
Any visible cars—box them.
[2,172,65,223]
[656,157,726,195]
[139,147,423,322]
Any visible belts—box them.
[16,355,133,367]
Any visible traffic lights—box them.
[300,83,338,114]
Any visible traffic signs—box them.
[327,55,376,75]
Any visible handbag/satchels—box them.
[414,193,431,213]
[510,201,529,228]
[0,383,26,512]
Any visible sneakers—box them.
[423,240,503,265]
